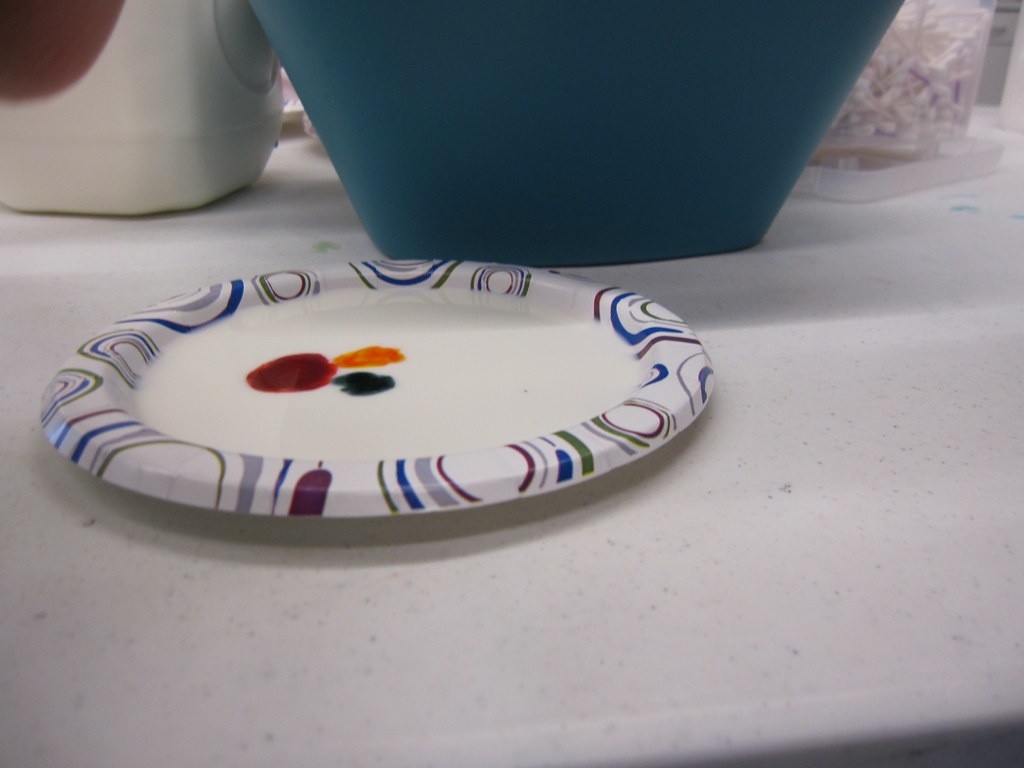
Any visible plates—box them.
[42,257,717,521]
